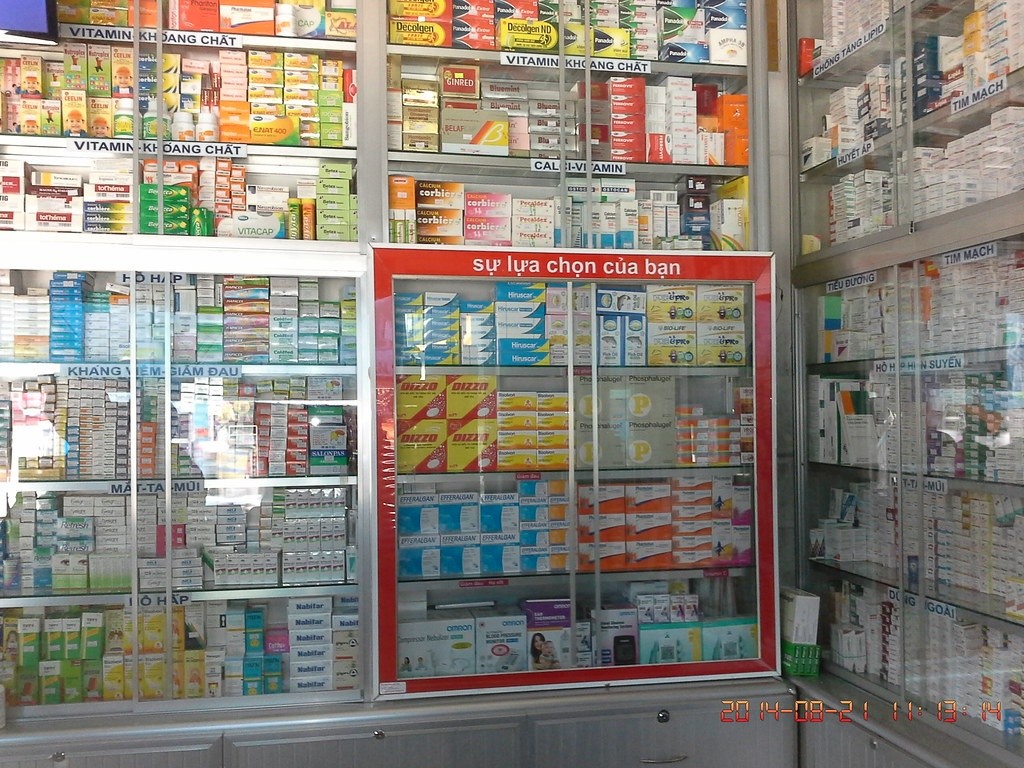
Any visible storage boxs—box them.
[1,1,1024,768]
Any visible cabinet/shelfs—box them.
[0,0,798,768]
[777,0,1024,768]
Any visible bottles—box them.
[112,98,218,141]
[274,3,298,36]
